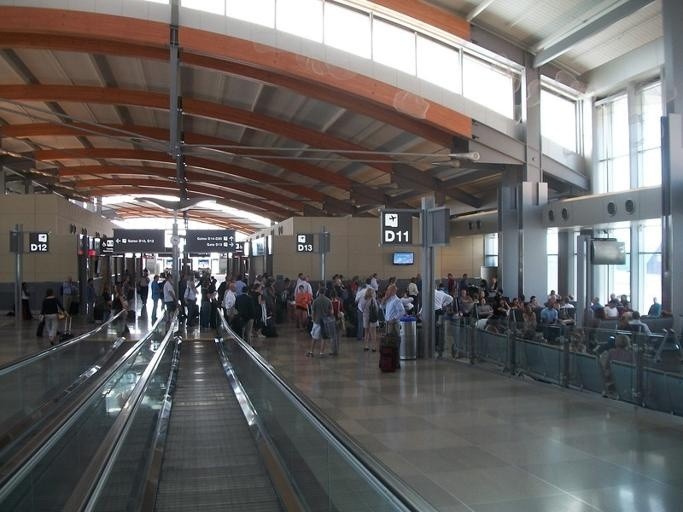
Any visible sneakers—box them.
[306,350,329,358]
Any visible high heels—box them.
[362,344,377,351]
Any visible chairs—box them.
[449,287,683,417]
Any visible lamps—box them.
[176,95,183,112]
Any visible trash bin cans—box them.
[399,316,417,359]
[443,317,461,350]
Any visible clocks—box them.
[170,235,180,246]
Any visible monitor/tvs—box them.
[393,251,414,265]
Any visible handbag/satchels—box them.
[337,311,346,332]
[370,299,378,323]
[311,323,322,340]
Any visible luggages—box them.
[379,320,400,373]
[59,315,74,344]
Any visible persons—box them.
[281,272,422,358]
[20,270,151,344]
[433,272,673,360]
[150,270,280,347]
